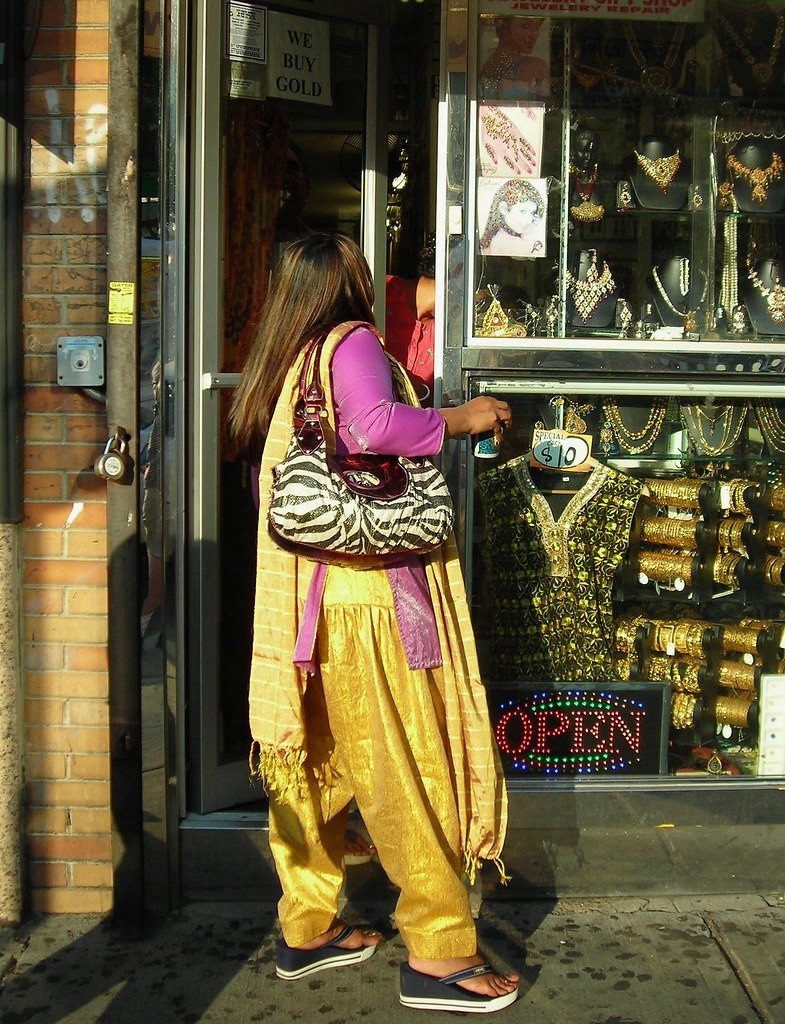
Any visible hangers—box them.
[474,395,651,498]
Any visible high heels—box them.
[400,960,519,1014]
[274,925,376,980]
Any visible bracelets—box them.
[616,476,784,730]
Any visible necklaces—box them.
[553,248,616,324]
[726,150,785,210]
[711,6,785,84]
[676,397,749,456]
[651,257,708,333]
[753,398,785,453]
[719,215,739,330]
[632,145,680,197]
[570,164,605,222]
[601,396,670,456]
[745,241,785,325]
[621,20,686,96]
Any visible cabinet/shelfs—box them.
[430,0,785,781]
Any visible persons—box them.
[569,129,604,204]
[479,17,551,99]
[221,234,522,1013]
[386,228,533,409]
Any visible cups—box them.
[472,421,503,459]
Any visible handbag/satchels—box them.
[266,320,455,571]
